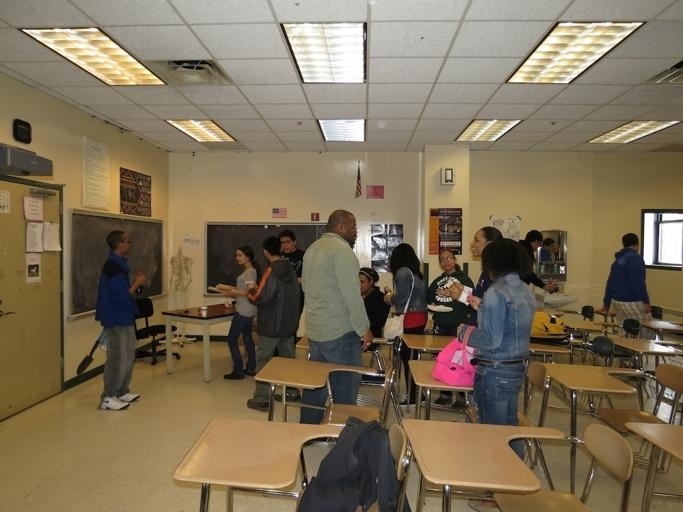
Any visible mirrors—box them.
[535,230,567,282]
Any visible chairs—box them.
[582,305,596,321]
[650,304,664,320]
[569,338,615,368]
[348,425,412,512]
[492,423,635,512]
[594,363,683,474]
[327,336,408,425]
[622,317,642,340]
[130,297,181,366]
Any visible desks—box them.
[400,332,468,361]
[612,338,682,368]
[620,417,682,512]
[295,334,387,360]
[168,414,345,512]
[638,318,682,341]
[399,417,570,512]
[538,362,643,494]
[564,321,620,338]
[253,357,377,421]
[407,357,478,420]
[531,331,566,342]
[160,302,238,382]
[523,343,572,415]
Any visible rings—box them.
[366,343,371,345]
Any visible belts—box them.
[478,359,522,365]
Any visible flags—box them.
[355,164,363,199]
[271,207,288,219]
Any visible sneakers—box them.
[248,399,268,412]
[100,393,140,410]
[451,402,468,409]
[224,369,256,379]
[435,395,451,405]
[275,393,301,402]
[394,393,426,407]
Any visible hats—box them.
[359,267,379,282]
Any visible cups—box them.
[200,306,207,317]
[133,271,144,293]
[225,296,233,308]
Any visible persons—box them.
[359,266,391,352]
[298,210,373,426]
[456,237,537,462]
[223,245,259,380]
[93,230,148,411]
[540,236,555,273]
[450,226,504,329]
[596,232,653,367]
[426,248,476,410]
[382,242,428,407]
[518,229,558,294]
[247,236,301,412]
[276,229,306,313]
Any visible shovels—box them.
[76,285,144,375]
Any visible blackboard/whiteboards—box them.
[203,221,330,298]
[66,208,168,323]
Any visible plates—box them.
[427,304,453,313]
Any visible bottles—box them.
[382,285,391,295]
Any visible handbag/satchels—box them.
[532,310,564,336]
[433,336,476,387]
[384,312,404,339]
